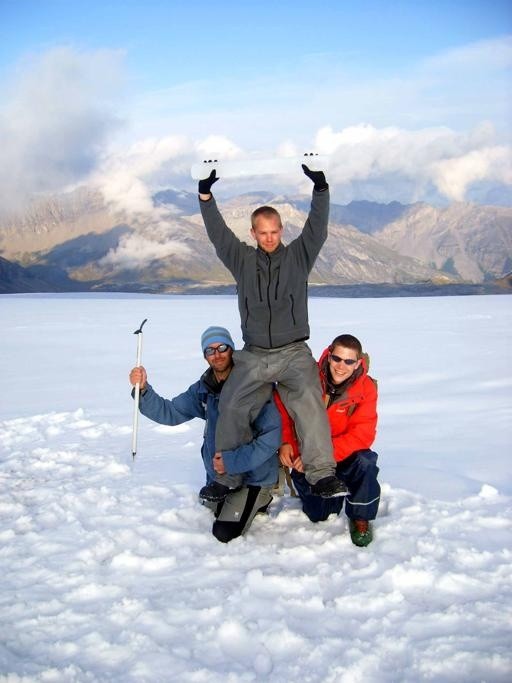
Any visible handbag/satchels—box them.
[140,387,145,390]
[281,441,288,446]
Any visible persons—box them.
[196,152,353,500]
[271,333,381,547]
[130,324,282,544]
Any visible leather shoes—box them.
[198,159,220,194]
[301,152,327,190]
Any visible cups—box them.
[205,344,230,356]
[330,354,356,365]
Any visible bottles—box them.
[199,326,235,353]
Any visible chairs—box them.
[199,478,243,501]
[349,515,373,547]
[309,477,351,499]
